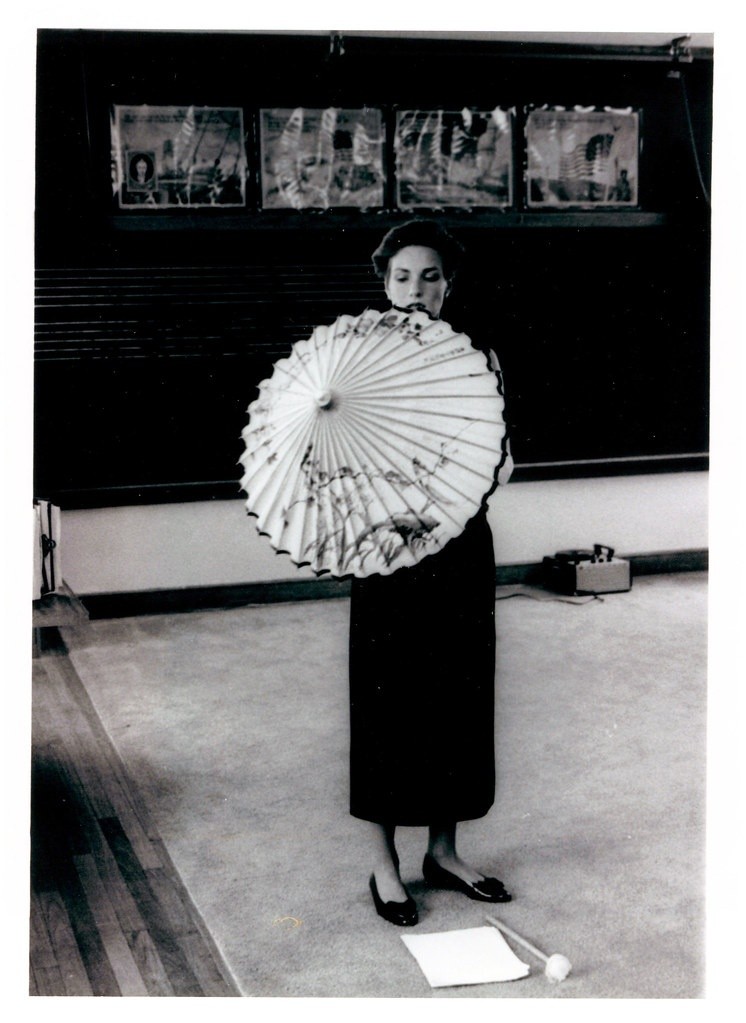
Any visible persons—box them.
[344,218,512,929]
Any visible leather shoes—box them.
[369,874,419,927]
[422,853,512,903]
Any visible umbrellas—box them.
[237,305,512,584]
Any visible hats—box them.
[372,219,464,285]
